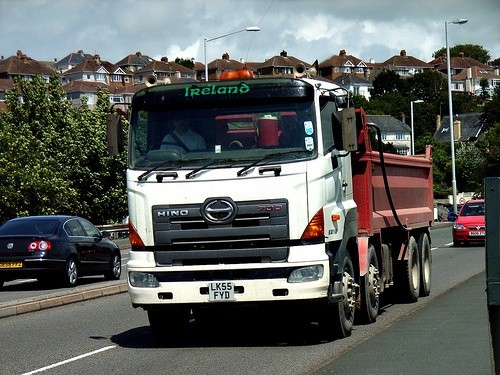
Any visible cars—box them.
[448,199,485,246]
[0,215,122,289]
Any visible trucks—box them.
[105,63,435,339]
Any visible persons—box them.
[161,113,206,151]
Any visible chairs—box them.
[208,118,228,148]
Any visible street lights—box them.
[411,99,425,155]
[444,17,468,208]
[203,27,261,82]
